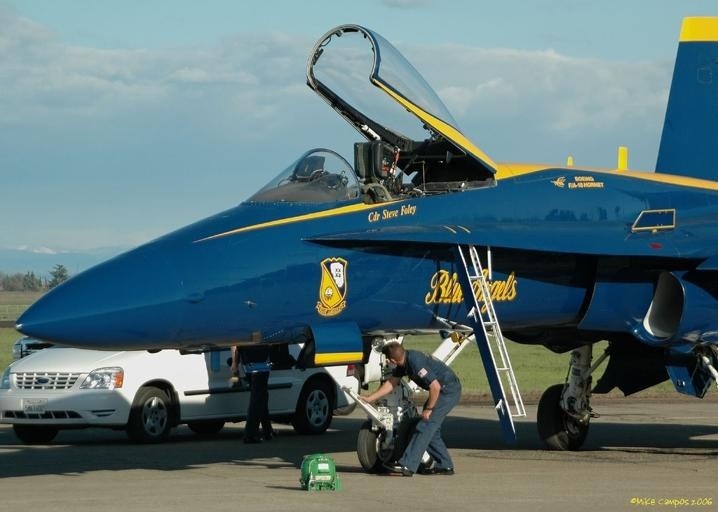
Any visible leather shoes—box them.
[383,461,413,477]
[422,467,453,475]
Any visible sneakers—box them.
[244,433,272,443]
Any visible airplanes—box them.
[15,16,717,472]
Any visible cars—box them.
[0,344,360,445]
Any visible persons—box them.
[357,343,461,475]
[230,343,279,443]
